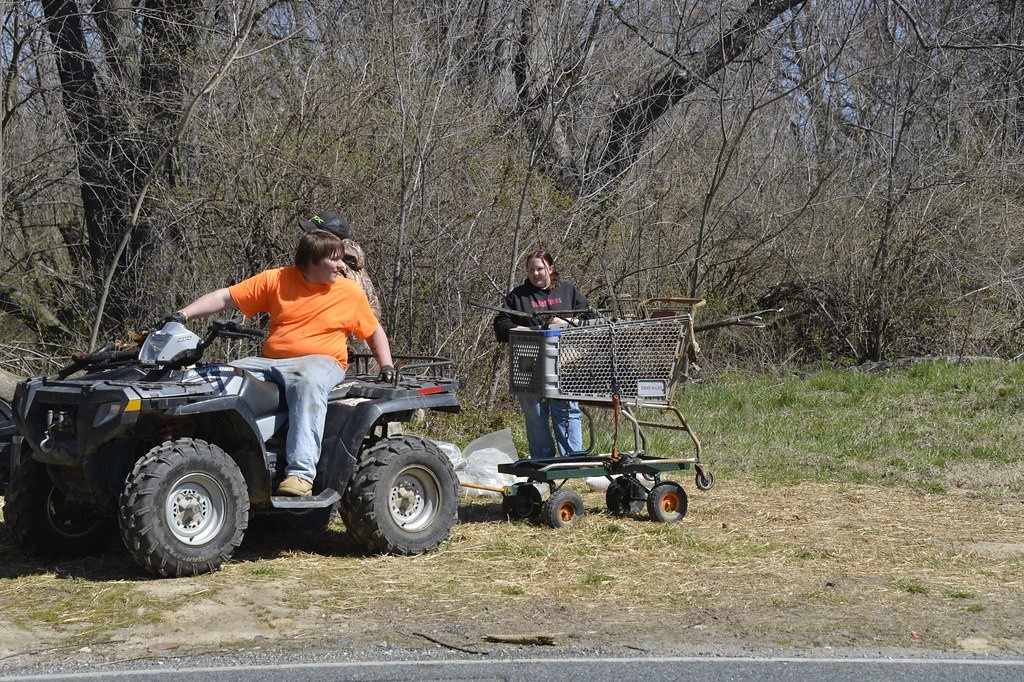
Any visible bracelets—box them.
[178,310,188,321]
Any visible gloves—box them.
[377,365,405,383]
[157,312,188,330]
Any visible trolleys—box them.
[507,295,715,491]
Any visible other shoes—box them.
[277,476,313,497]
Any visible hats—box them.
[298,211,348,239]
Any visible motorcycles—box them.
[1,317,460,576]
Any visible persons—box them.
[298,210,381,377]
[137,229,398,494]
[493,250,592,492]
